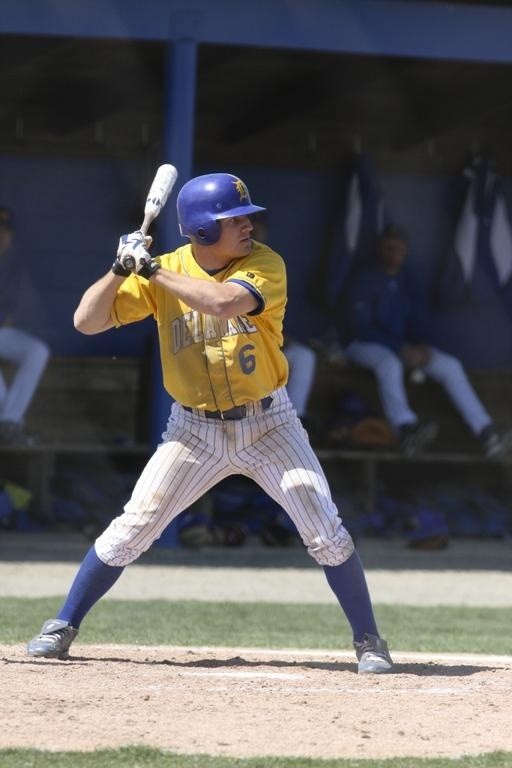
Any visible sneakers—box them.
[396,417,439,460]
[353,633,393,675]
[28,618,78,658]
[0,422,42,446]
[481,430,511,465]
[180,520,289,547]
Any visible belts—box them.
[182,395,272,421]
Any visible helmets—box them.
[176,173,266,246]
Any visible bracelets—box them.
[112,258,131,277]
[137,257,159,279]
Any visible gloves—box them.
[111,231,162,279]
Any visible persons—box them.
[327,224,511,463]
[27,172,395,675]
[248,217,320,446]
[0,209,51,445]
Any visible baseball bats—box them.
[123,164,178,270]
